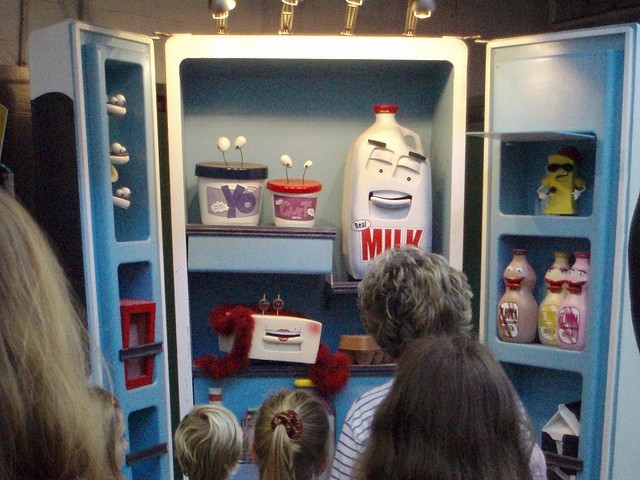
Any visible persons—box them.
[351,337,536,479]
[173,404,245,480]
[1,188,116,479]
[249,390,334,480]
[86,386,129,478]
[329,245,548,478]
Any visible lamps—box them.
[278,0,298,35]
[341,1,363,36]
[402,0,436,37]
[207,0,237,35]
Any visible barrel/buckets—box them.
[342,104,432,282]
